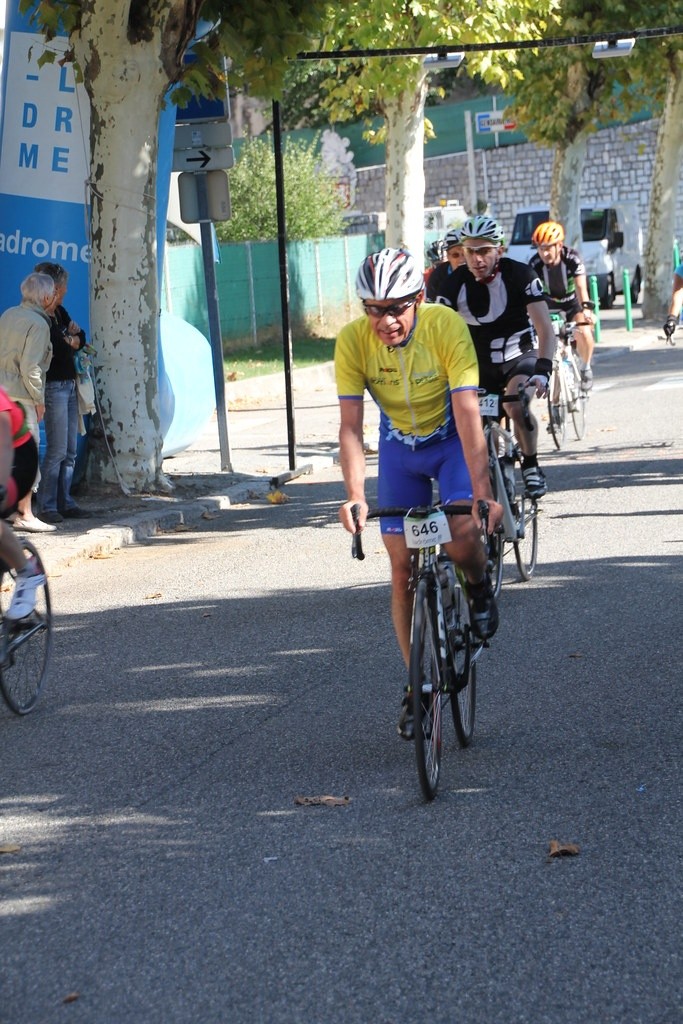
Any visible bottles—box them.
[434,563,454,625]
[444,560,459,611]
[501,456,515,504]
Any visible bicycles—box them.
[477,383,544,598]
[0,537,55,715]
[547,322,590,450]
[351,500,491,801]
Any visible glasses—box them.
[54,265,62,280]
[362,297,416,318]
[462,242,501,257]
[446,251,464,258]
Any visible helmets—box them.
[355,247,425,300]
[532,222,564,246]
[442,228,462,252]
[427,240,446,261]
[459,215,504,246]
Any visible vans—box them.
[503,199,643,308]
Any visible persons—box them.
[527,222,595,433]
[34,262,93,524]
[0,388,40,619]
[0,273,57,532]
[662,262,683,336]
[423,216,558,568]
[333,247,504,738]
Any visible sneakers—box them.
[546,416,560,431]
[397,676,429,740]
[6,572,46,622]
[521,462,540,492]
[5,512,21,523]
[465,570,499,640]
[581,369,593,391]
[63,507,92,518]
[13,517,57,532]
[41,511,63,522]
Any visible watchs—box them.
[67,335,72,345]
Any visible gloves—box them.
[663,315,679,337]
